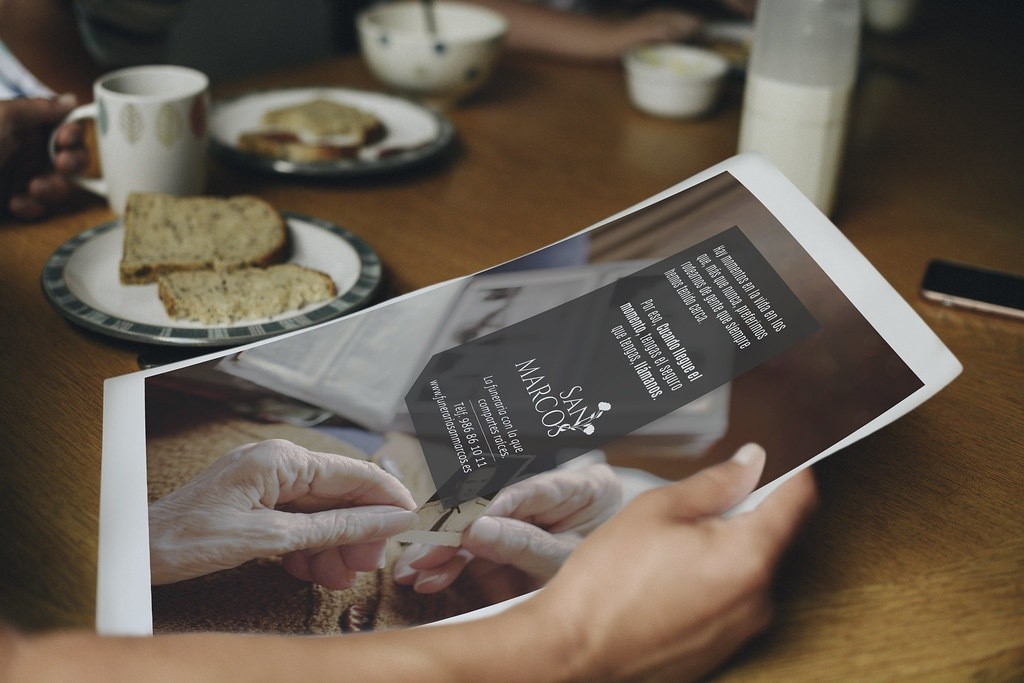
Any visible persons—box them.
[416,457,499,534]
[0,98,819,683]
[327,0,749,65]
[148,439,626,604]
[0,0,201,100]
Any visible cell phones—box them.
[920,258,1024,321]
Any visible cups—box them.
[50,64,211,216]
[737,2,861,230]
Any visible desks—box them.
[0,0,1024,682]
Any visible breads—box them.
[119,191,291,284]
[237,98,384,161]
[156,263,335,327]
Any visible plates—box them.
[41,210,382,345]
[210,88,454,177]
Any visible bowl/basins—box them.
[626,43,728,117]
[358,1,508,103]
